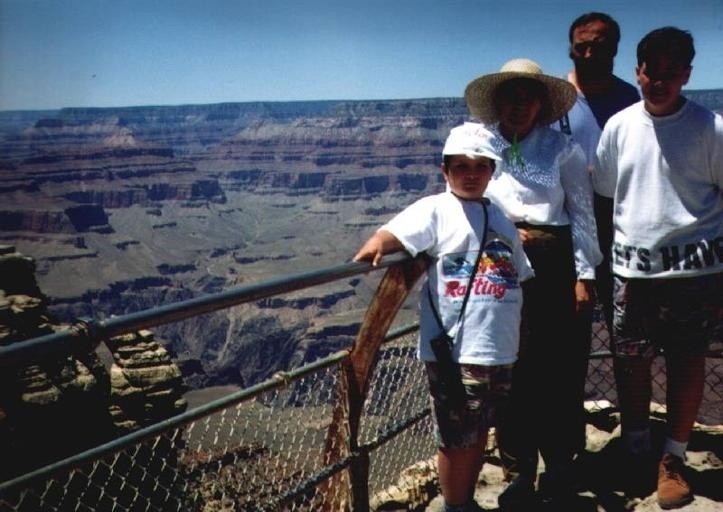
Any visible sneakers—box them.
[657,452,694,510]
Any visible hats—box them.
[463,59,578,128]
[442,123,504,163]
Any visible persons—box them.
[586,26,722,511]
[545,10,633,463]
[441,77,605,508]
[349,119,539,510]
